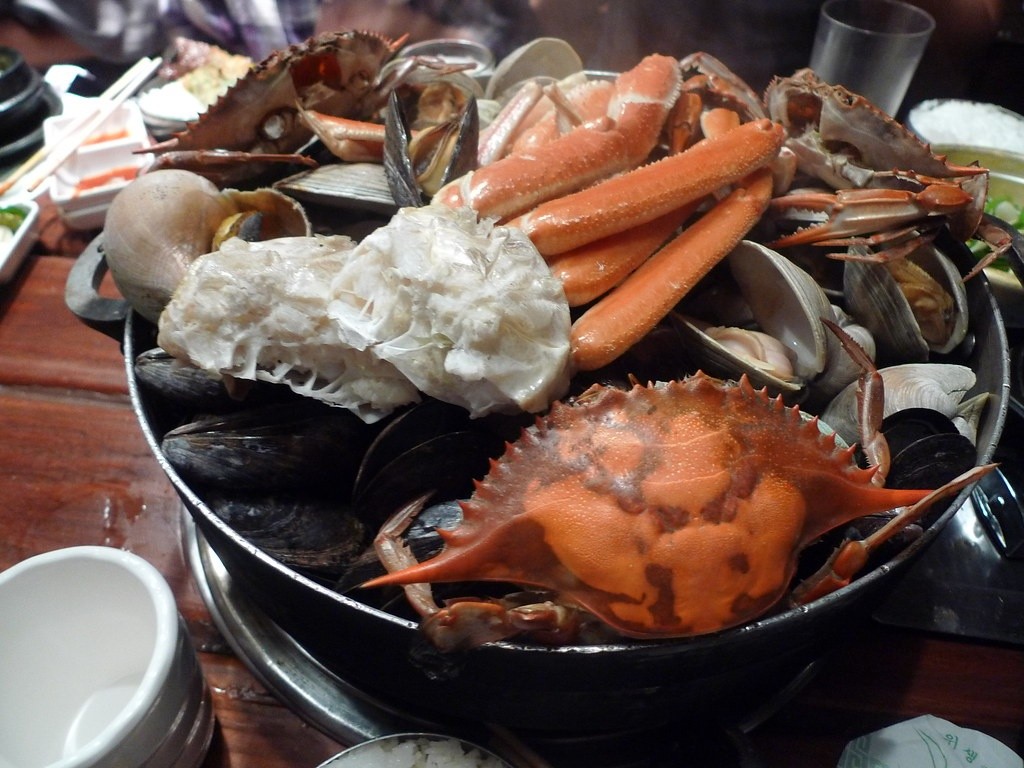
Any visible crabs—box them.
[131,30,483,181]
[356,364,1005,658]
[430,53,798,368]
[679,48,1024,289]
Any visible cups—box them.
[809,0,936,127]
[0,544,224,768]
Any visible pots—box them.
[64,95,1010,652]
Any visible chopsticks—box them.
[0,56,162,197]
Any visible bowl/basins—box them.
[48,138,157,210]
[57,202,113,230]
[43,99,147,172]
[140,89,207,138]
[926,144,1024,290]
[906,98,1024,154]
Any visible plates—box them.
[314,733,514,768]
